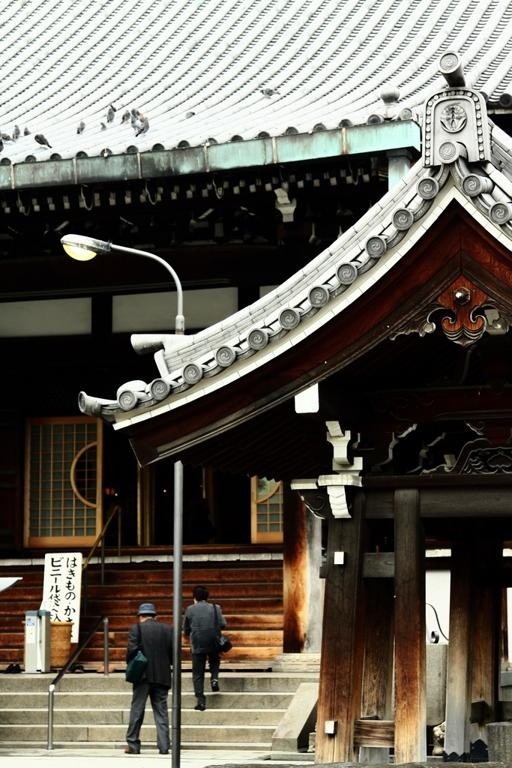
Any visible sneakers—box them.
[125,749,140,754]
[74,665,85,673]
[9,664,21,673]
[4,663,14,674]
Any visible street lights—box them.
[59,233,185,768]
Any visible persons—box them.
[183,586,233,710]
[124,603,173,754]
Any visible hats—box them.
[136,603,157,616]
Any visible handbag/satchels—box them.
[126,622,149,684]
[213,602,232,652]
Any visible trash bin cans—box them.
[23,610,51,673]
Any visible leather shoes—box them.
[210,681,219,692]
[195,703,206,710]
